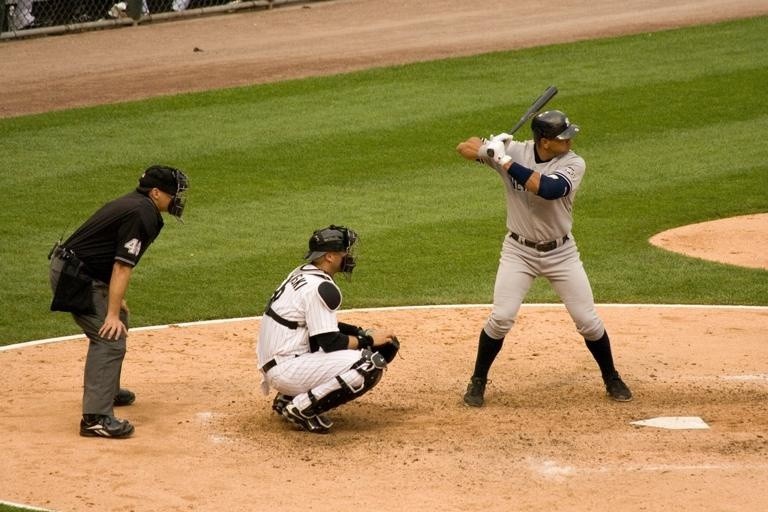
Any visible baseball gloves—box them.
[358,328,400,361]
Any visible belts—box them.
[56,247,92,275]
[509,231,568,253]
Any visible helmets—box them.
[137,165,190,217]
[531,110,580,140]
[304,225,359,282]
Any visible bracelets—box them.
[504,162,532,189]
[358,335,372,348]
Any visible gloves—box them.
[478,132,514,166]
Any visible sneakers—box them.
[272,392,293,414]
[80,415,133,437]
[464,374,487,407]
[283,401,329,434]
[114,389,135,406]
[602,371,633,402]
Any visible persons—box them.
[1,0,222,34]
[46,165,189,438]
[258,225,400,433]
[455,108,633,408]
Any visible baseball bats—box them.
[487,86,558,157]
